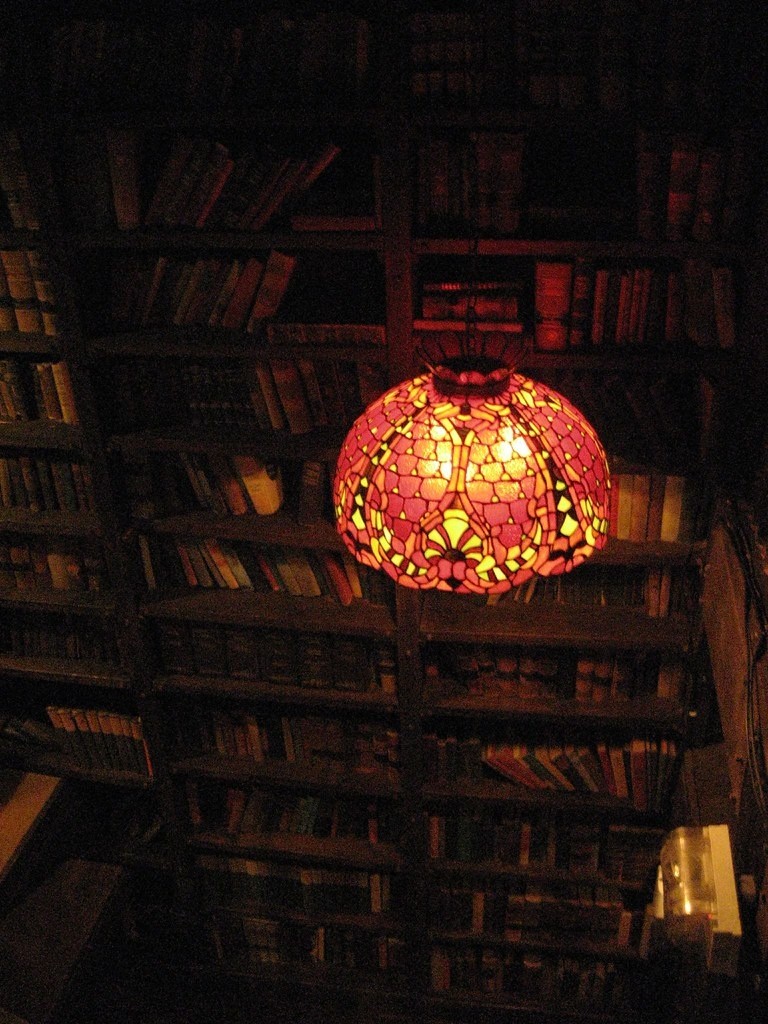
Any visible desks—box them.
[0,856,123,1024]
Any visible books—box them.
[0,0,768,1024]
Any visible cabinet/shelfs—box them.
[0,101,746,1024]
